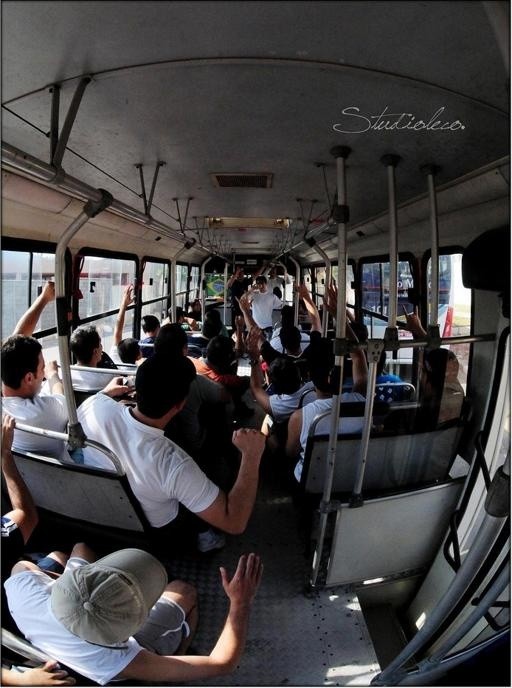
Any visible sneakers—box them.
[197,533,227,553]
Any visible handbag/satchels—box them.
[97,351,116,369]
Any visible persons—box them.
[76,349,271,556]
[1,412,39,560]
[2,660,77,686]
[4,538,267,686]
[2,259,463,475]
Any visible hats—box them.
[51,548,168,645]
